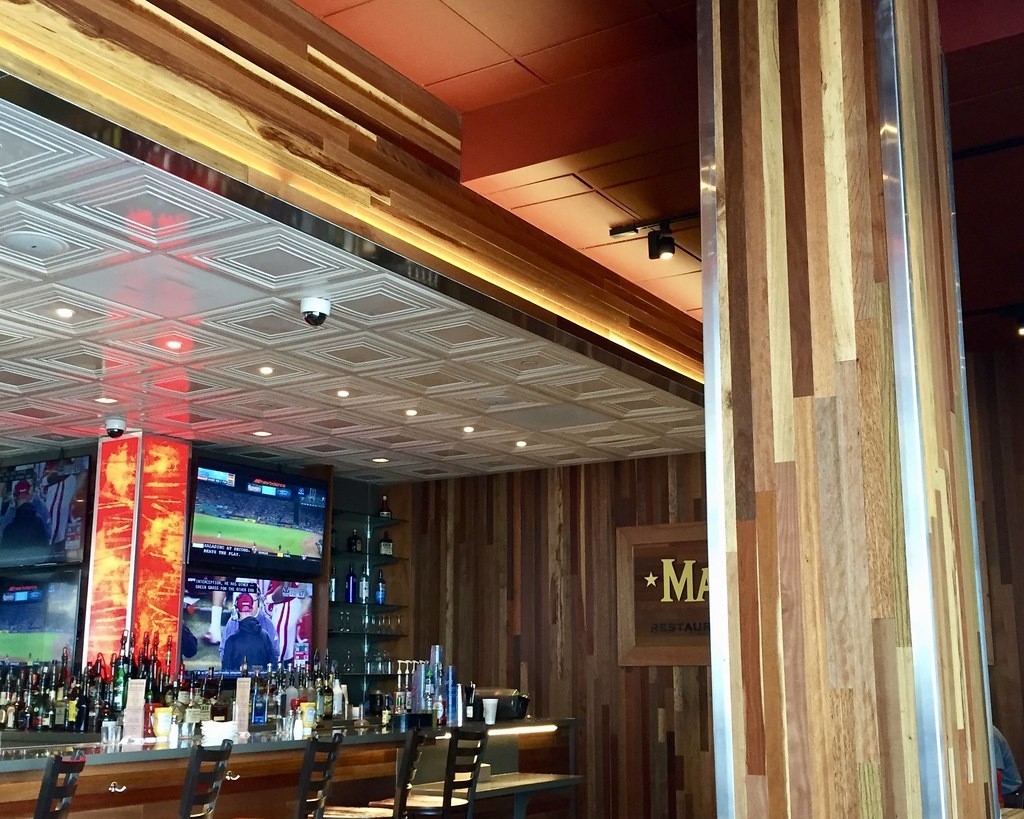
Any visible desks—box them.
[408,771,585,819]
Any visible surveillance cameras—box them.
[105,420,126,438]
[300,298,331,326]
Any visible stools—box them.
[34,749,86,819]
[234,734,344,819]
[308,724,427,819]
[178,739,232,819]
[368,725,487,819]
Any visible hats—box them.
[235,593,253,612]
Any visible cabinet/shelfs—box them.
[326,507,410,706]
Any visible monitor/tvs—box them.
[180,573,316,689]
[186,458,329,578]
[0,568,86,679]
[0,445,100,575]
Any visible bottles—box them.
[379,489,392,519]
[0,629,228,744]
[229,649,342,733]
[374,569,387,602]
[329,565,337,602]
[336,612,351,632]
[426,663,446,725]
[343,651,353,672]
[348,530,362,552]
[371,614,403,634]
[359,564,370,604]
[345,563,357,602]
[366,650,392,675]
[378,531,393,556]
[331,519,337,550]
[381,694,404,726]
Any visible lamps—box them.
[649,223,675,261]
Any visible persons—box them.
[217,529,285,557]
[0,456,85,561]
[180,577,312,675]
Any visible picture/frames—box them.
[615,522,711,667]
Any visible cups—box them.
[369,689,383,706]
[414,645,458,728]
[482,699,498,725]
[151,707,172,737]
